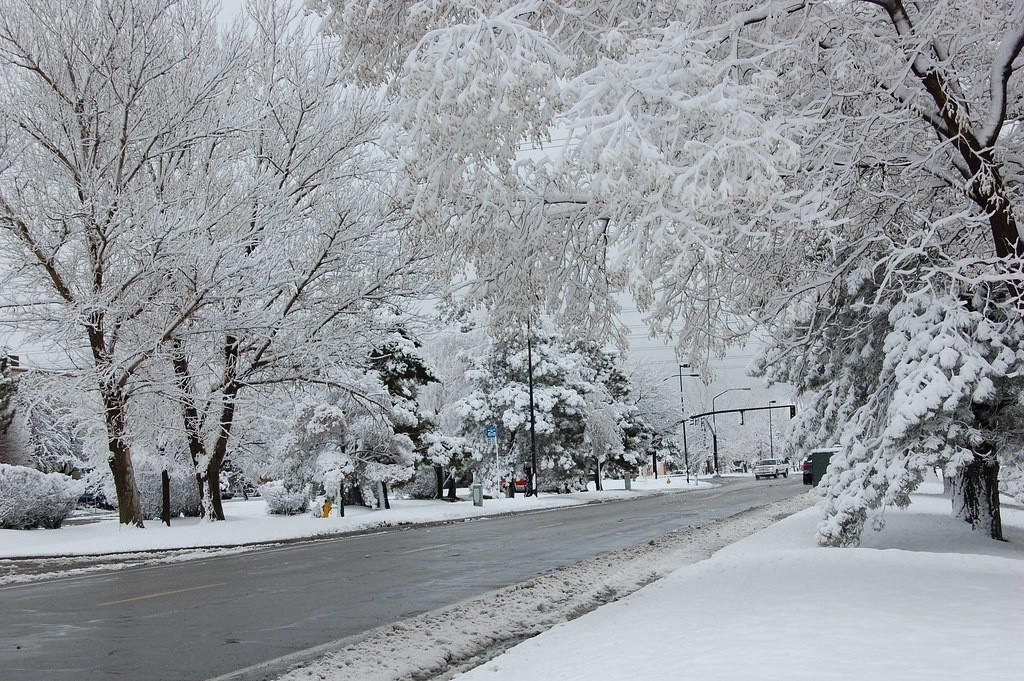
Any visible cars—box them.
[802,454,812,485]
[499,471,528,492]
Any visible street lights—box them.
[712,388,751,478]
[680,363,700,485]
[769,400,777,460]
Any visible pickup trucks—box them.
[754,458,790,479]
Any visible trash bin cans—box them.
[473,483,483,507]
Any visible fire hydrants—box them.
[322,500,333,518]
